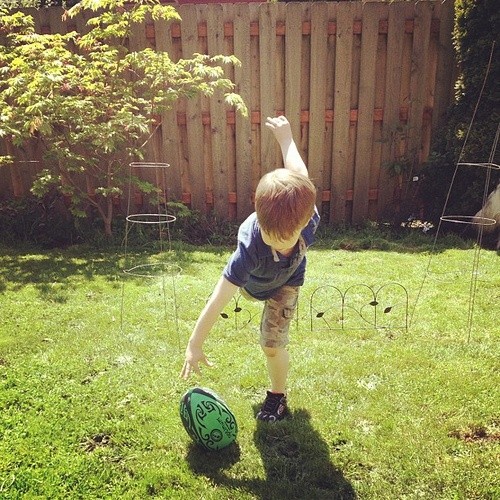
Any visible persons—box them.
[180,115,322,423]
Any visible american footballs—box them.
[180,387,238,452]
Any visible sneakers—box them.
[254,389,287,421]
